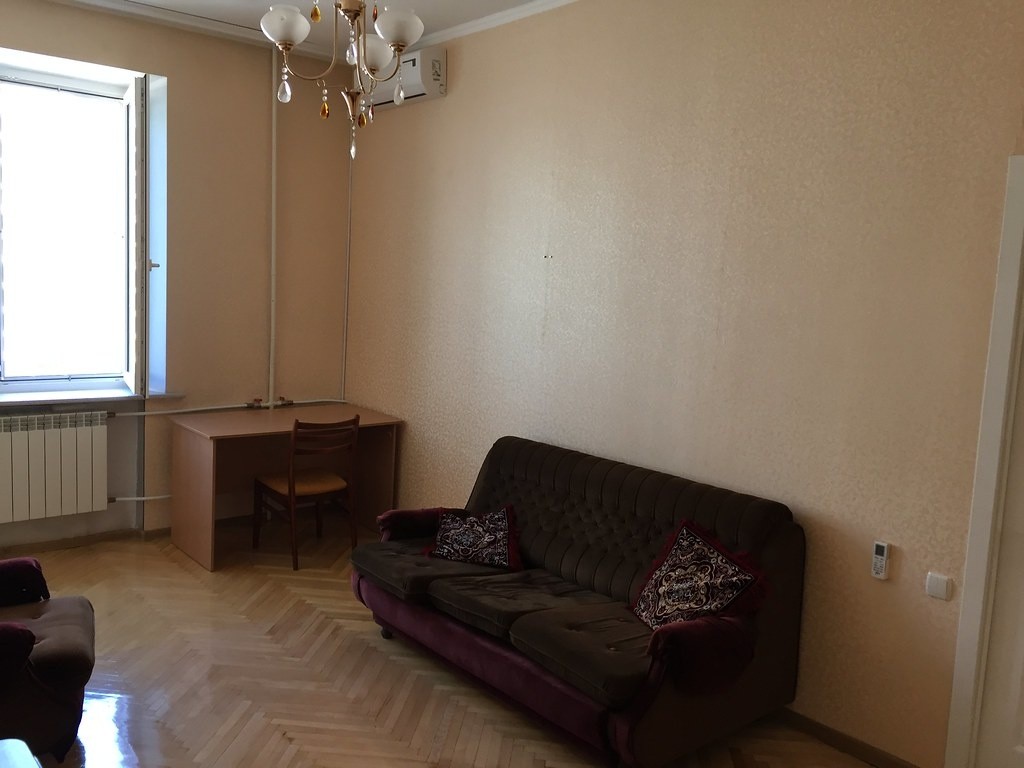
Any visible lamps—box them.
[260,0,427,159]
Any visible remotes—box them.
[872,541,889,579]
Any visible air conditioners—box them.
[354,47,451,111]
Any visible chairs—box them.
[251,415,360,571]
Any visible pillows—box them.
[633,515,774,637]
[428,506,524,568]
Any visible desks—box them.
[168,396,406,572]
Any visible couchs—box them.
[0,559,95,756]
[349,433,805,768]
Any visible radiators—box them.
[1,414,106,524]
